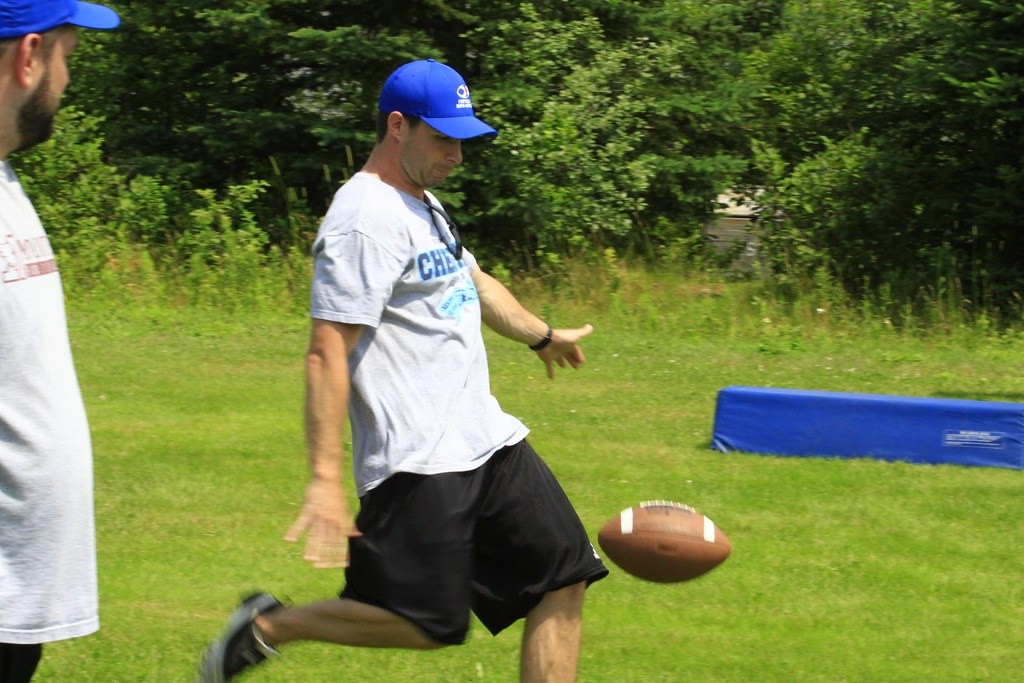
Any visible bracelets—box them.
[529,326,553,351]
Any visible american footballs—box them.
[599,500,732,583]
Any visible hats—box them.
[0,0,120,37]
[378,59,498,140]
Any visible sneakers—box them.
[203,591,283,682]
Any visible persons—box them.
[208,59,609,683]
[0,0,121,678]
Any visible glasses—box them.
[428,204,462,260]
